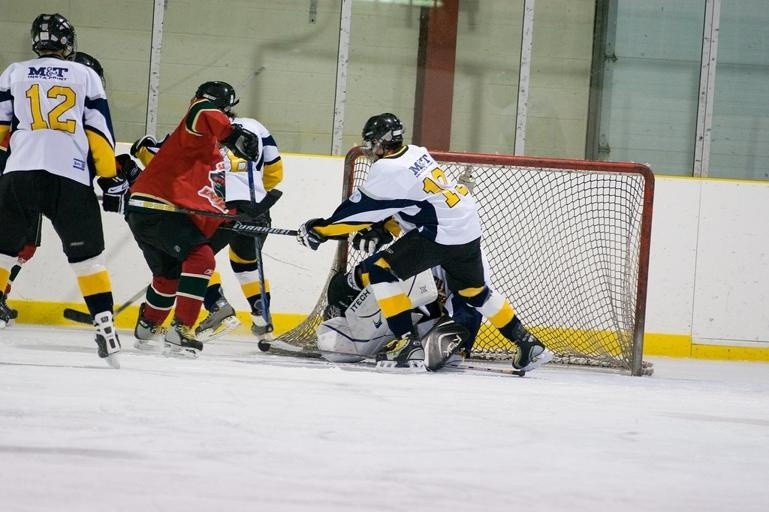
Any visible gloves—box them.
[297,217,328,252]
[98,170,131,213]
[230,126,259,161]
[130,135,158,156]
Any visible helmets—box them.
[195,80,239,113]
[30,13,75,57]
[362,112,405,151]
[68,51,105,88]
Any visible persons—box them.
[118,81,259,353]
[315,211,492,373]
[2,10,127,359]
[132,83,284,340]
[298,112,554,373]
[0,45,118,320]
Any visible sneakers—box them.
[165,319,203,350]
[195,300,236,335]
[375,333,425,361]
[512,334,544,368]
[94,310,121,357]
[135,319,167,340]
[250,319,274,335]
[0,290,17,320]
